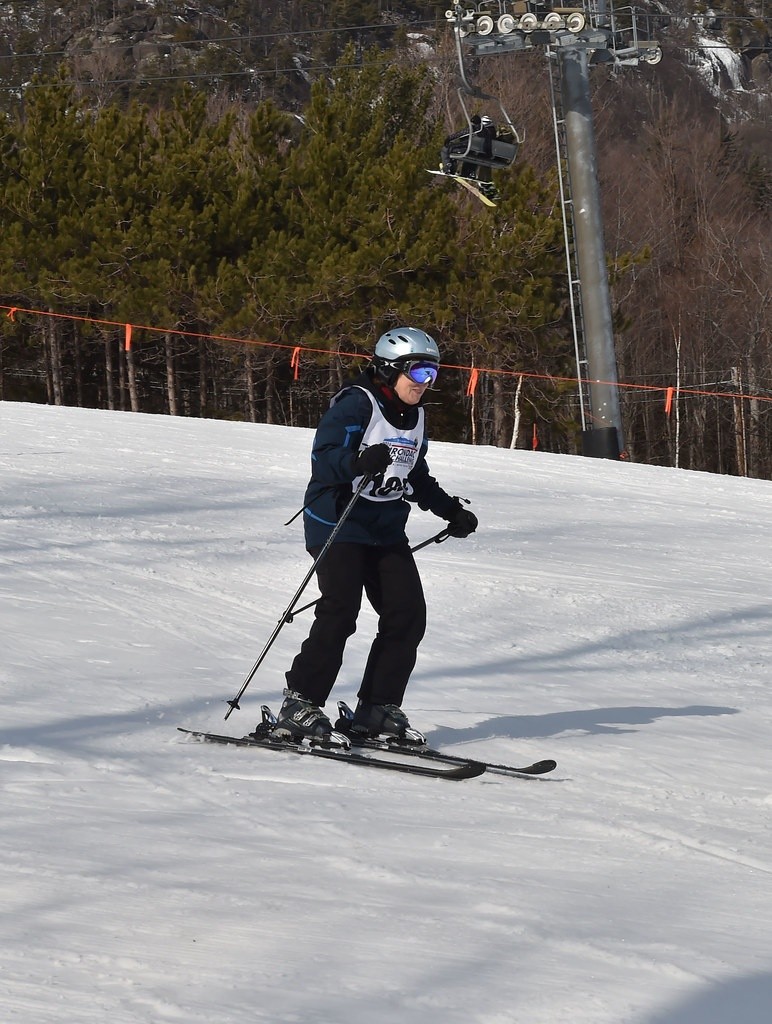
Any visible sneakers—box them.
[274,687,351,752]
[350,697,428,747]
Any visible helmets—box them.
[373,327,440,363]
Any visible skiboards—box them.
[178,706,558,781]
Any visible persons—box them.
[275,328,477,743]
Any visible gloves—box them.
[446,507,478,539]
[357,443,393,476]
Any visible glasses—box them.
[401,361,438,385]
